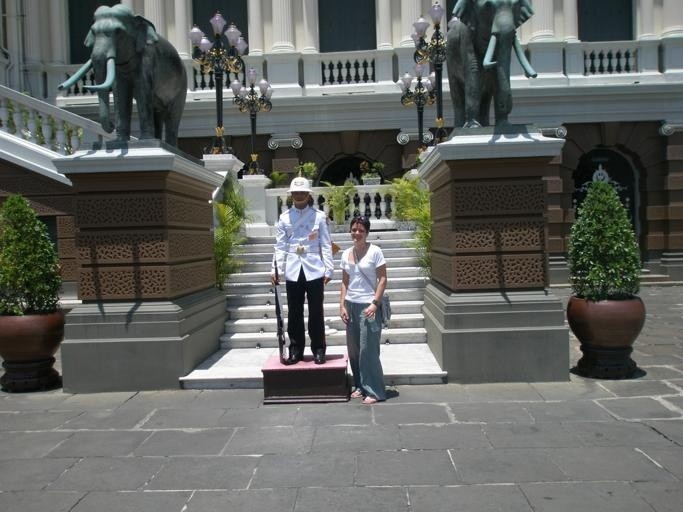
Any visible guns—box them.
[274,246,288,363]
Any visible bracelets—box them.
[372,300,381,310]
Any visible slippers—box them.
[362,396,378,404]
[350,391,363,398]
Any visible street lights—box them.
[397,64,436,151]
[191,11,246,155]
[411,1,447,145]
[232,68,273,175]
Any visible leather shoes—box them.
[313,354,326,364]
[286,353,304,364]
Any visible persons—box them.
[267,176,336,364]
[337,215,389,405]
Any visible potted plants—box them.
[566,179,645,376]
[1,191,64,391]
[303,161,425,232]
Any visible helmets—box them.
[287,177,313,193]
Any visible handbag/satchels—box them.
[381,294,392,328]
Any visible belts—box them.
[288,247,320,253]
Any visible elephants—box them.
[445,0,537,128]
[56,3,188,148]
